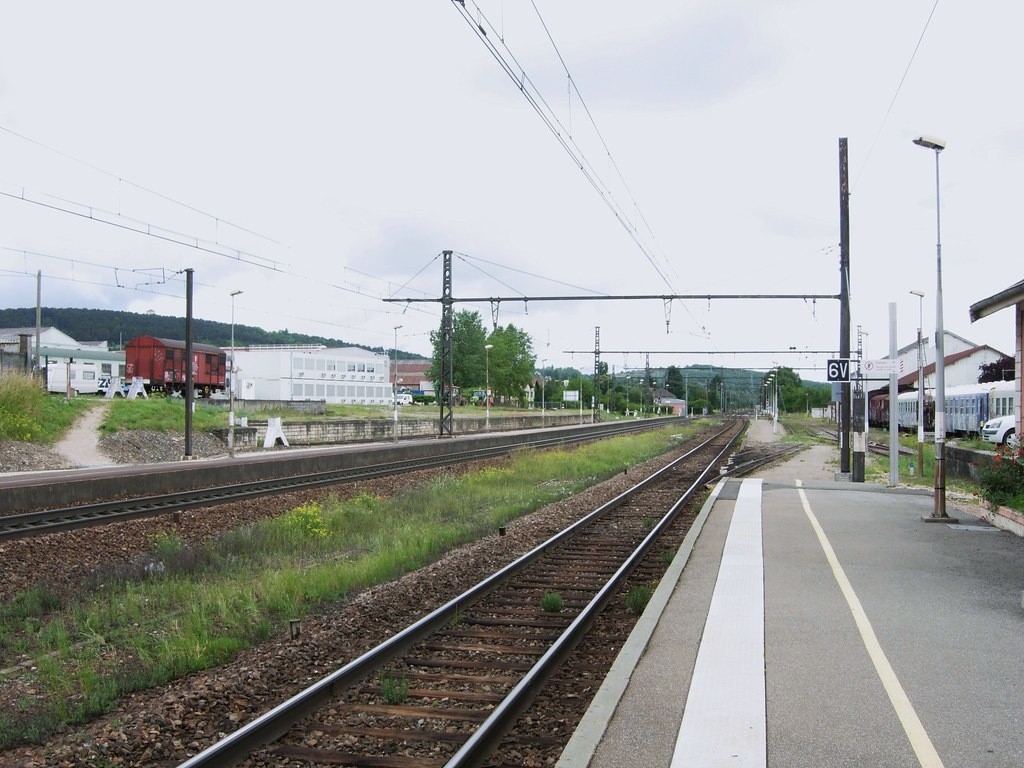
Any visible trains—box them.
[126,336,227,398]
[868,380,1014,439]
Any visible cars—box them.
[982,414,1015,449]
